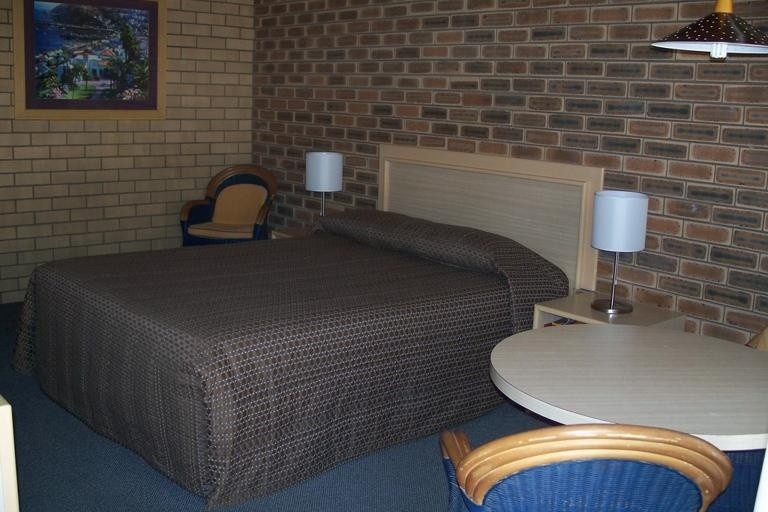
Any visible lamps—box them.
[588,189,650,318]
[303,149,344,215]
[644,0,768,62]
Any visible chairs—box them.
[434,422,735,511]
[703,322,768,512]
[179,162,281,250]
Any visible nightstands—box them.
[529,289,690,334]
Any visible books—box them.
[544,317,585,328]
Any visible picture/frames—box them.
[10,1,168,124]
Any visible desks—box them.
[486,322,767,511]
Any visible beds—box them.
[10,142,606,510]
